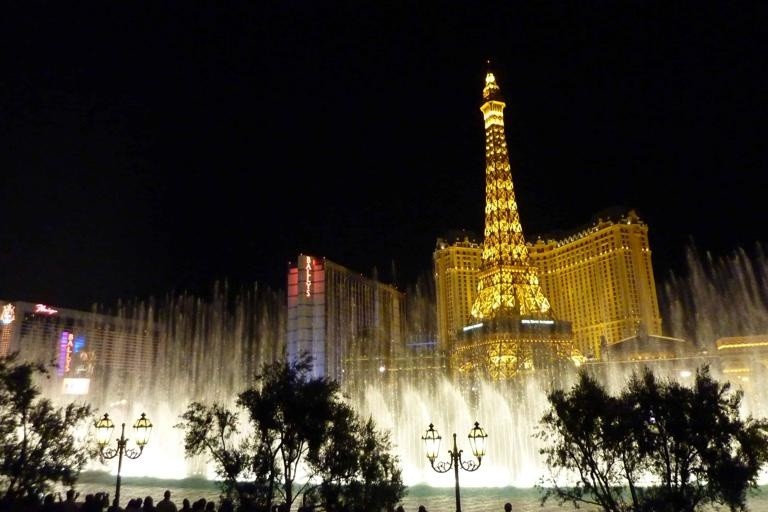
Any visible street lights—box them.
[421,421,489,512]
[94,412,153,500]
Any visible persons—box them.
[504,502,513,511]
[0,487,430,510]
